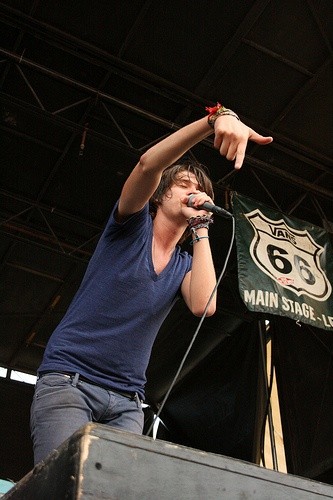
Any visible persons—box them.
[32,102,274,467]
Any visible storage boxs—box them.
[0,423,333,500]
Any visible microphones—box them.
[187,194,233,218]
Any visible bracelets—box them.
[208,108,240,129]
[189,216,210,245]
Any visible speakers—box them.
[0,422,333,500]
